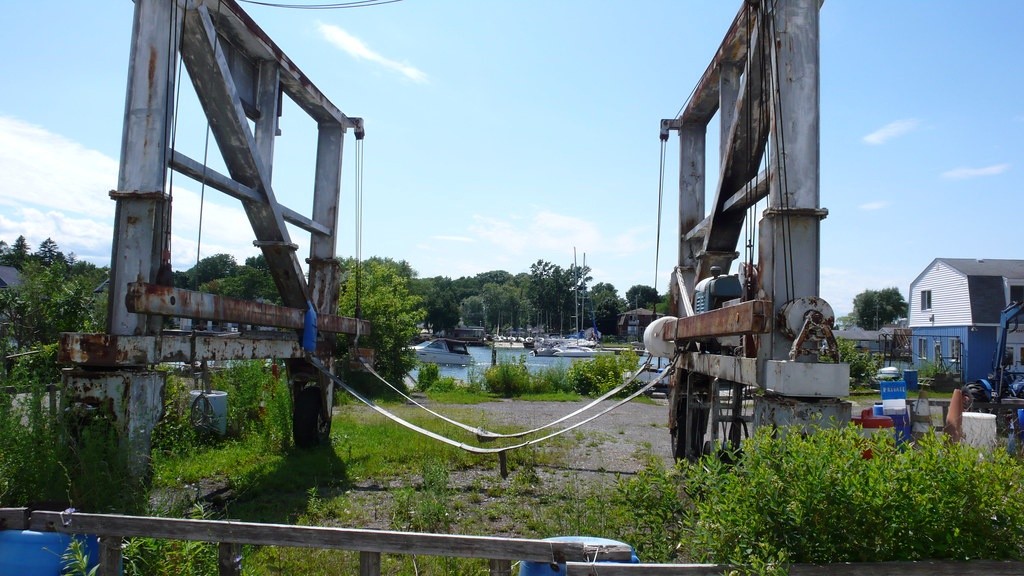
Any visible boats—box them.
[399,335,473,366]
[480,246,616,357]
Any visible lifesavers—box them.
[525,336,534,343]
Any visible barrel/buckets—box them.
[0,529,101,576]
[962,412,997,462]
[904,370,917,392]
[520,536,639,576]
[851,408,894,460]
[873,401,910,451]
